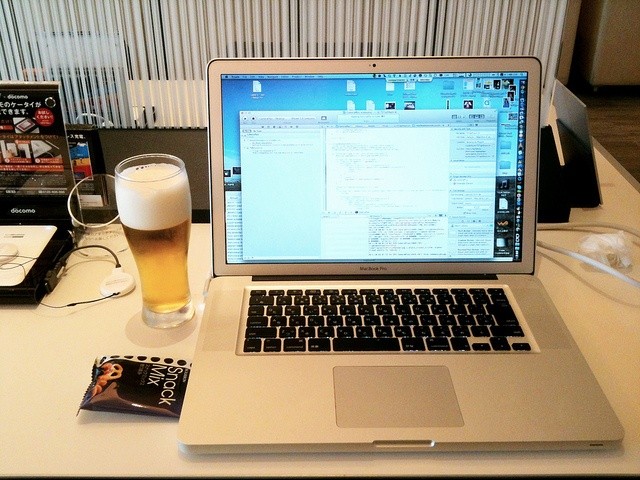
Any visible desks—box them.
[0,80,639,477]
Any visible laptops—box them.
[3,79,86,307]
[178,55,626,453]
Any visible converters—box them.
[99,266,136,300]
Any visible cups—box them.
[114,152,196,331]
